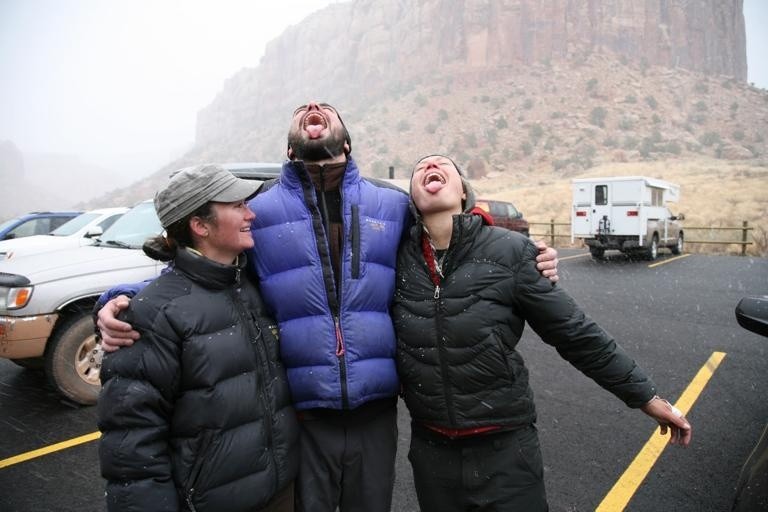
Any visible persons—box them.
[94,162,304,512]
[91,98,561,512]
[384,154,694,512]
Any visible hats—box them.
[153,162,266,230]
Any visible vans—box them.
[477,200,529,238]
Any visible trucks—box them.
[571,176,684,261]
